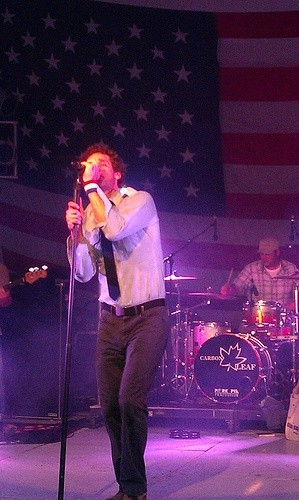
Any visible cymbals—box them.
[271,271,299,280]
[164,271,201,284]
[188,286,238,302]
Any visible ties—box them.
[98,200,120,301]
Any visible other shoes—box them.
[106,488,147,500]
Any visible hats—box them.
[256,238,281,254]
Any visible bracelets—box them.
[85,188,97,196]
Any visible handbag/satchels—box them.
[285,394,299,441]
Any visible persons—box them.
[66,144,169,500]
[0,264,12,306]
[221,238,299,310]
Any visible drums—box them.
[191,333,273,406]
[241,299,284,329]
[1,261,51,308]
[171,320,235,370]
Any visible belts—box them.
[99,299,167,317]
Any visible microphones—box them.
[212,220,218,240]
[290,216,294,241]
[250,279,259,297]
